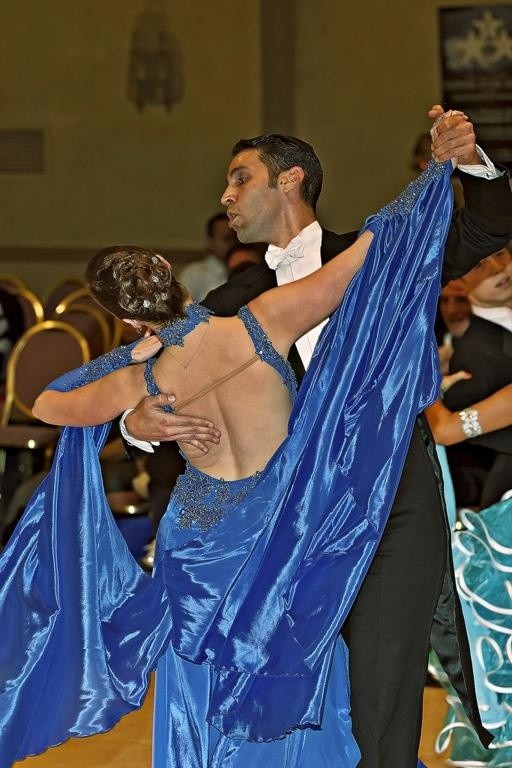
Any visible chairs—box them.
[0,272,149,539]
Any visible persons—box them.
[147,212,512,682]
[34,109,468,768]
[120,102,511,768]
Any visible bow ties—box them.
[265,235,305,270]
